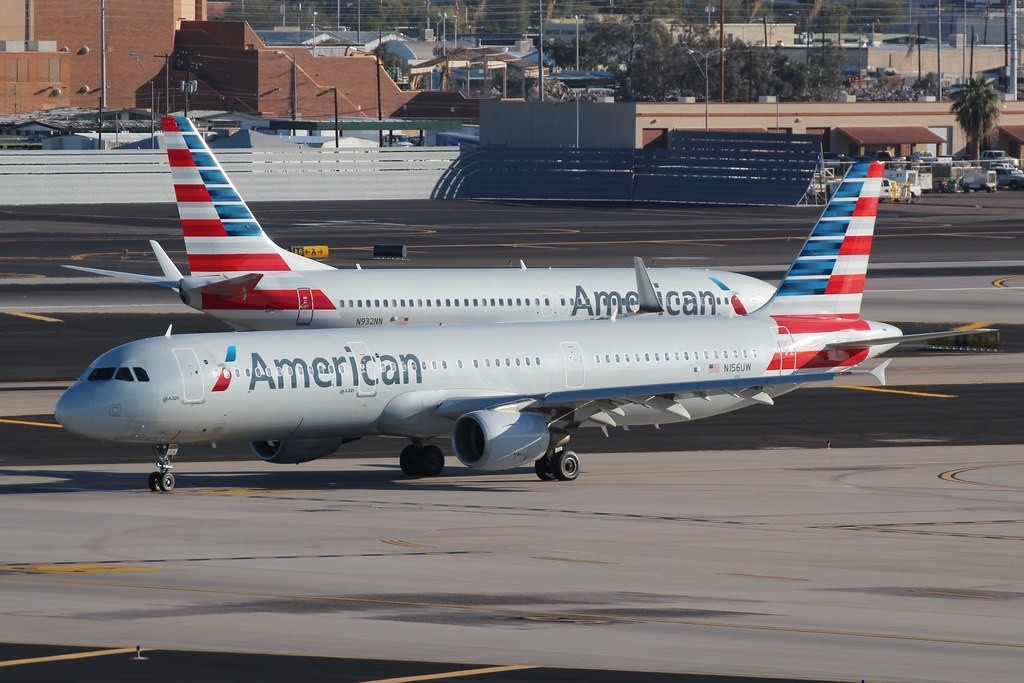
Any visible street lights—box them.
[555,82,579,147]
[274,50,296,136]
[128,52,155,149]
[687,47,726,131]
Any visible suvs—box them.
[992,166,1024,192]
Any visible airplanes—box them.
[53,116,1009,494]
